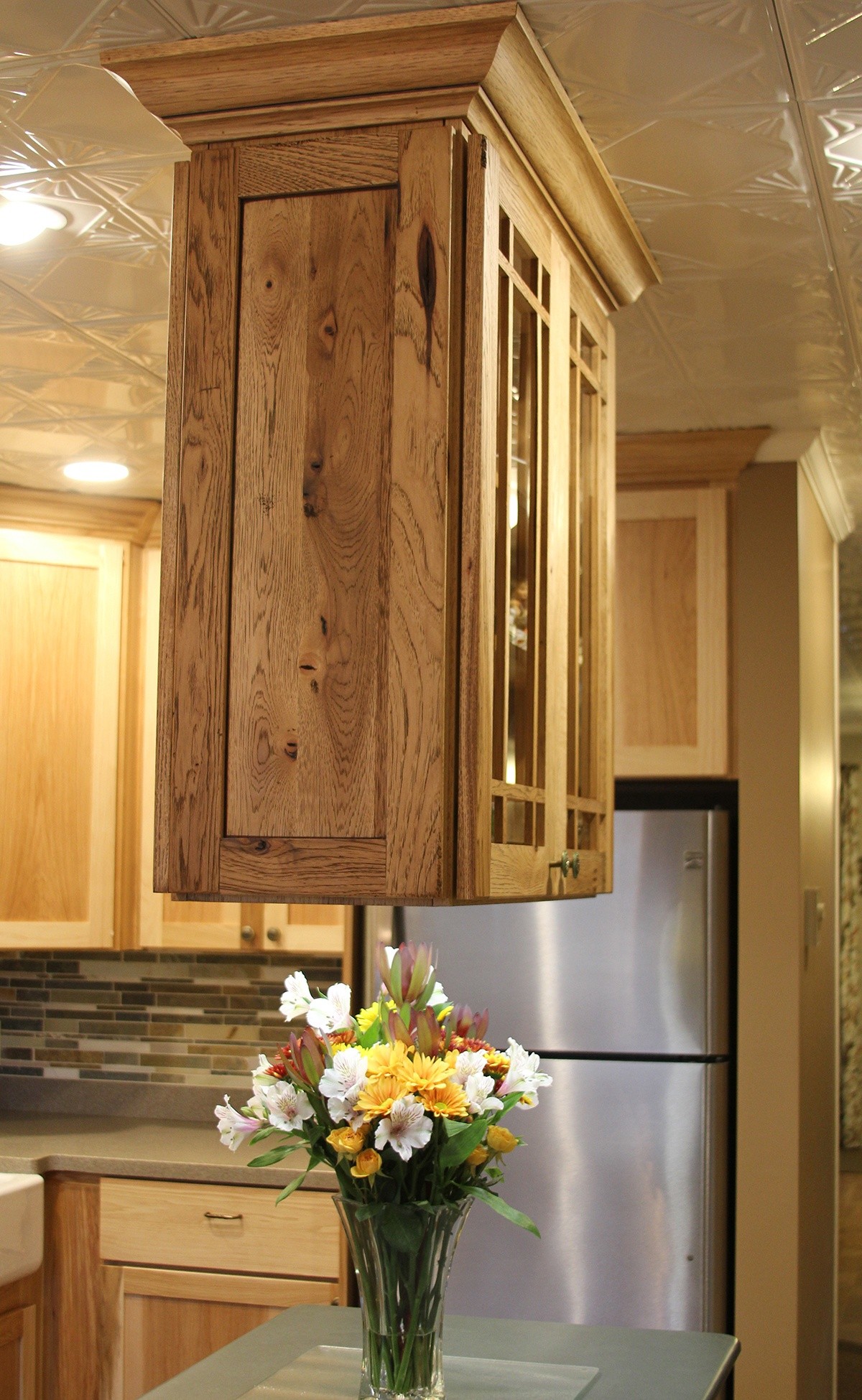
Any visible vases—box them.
[322,1194,475,1400]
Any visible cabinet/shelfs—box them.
[609,418,778,788]
[93,0,664,923]
[48,1181,351,1400]
[0,479,158,956]
[0,1251,53,1400]
[135,487,343,959]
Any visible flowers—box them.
[212,937,555,1392]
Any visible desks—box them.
[137,1300,742,1400]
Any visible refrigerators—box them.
[365,806,735,1330]
